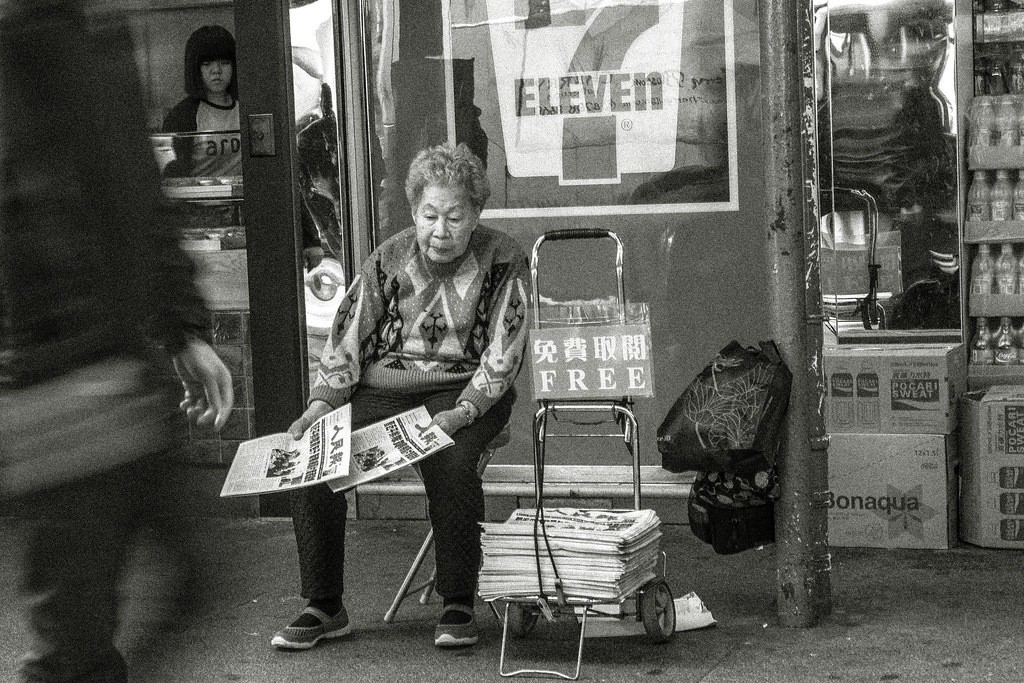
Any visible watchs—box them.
[456,404,474,426]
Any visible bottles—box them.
[970,317,1024,365]
[973,0,1024,94]
[973,244,1024,294]
[965,172,1024,222]
[972,99,1024,146]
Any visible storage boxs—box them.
[967,363,1024,391]
[822,344,967,435]
[964,208,1024,244]
[972,0,1024,44]
[968,280,1023,317]
[826,432,961,550]
[967,125,1024,171]
[958,385,1024,549]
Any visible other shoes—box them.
[434,603,478,646]
[271,602,351,649]
[15,644,128,683]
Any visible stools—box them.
[383,423,510,631]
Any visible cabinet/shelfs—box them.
[146,127,254,316]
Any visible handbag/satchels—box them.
[656,341,792,475]
[688,471,782,555]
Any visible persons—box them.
[271,142,533,649]
[0,1,245,683]
[297,84,342,237]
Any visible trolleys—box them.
[483,228,677,682]
